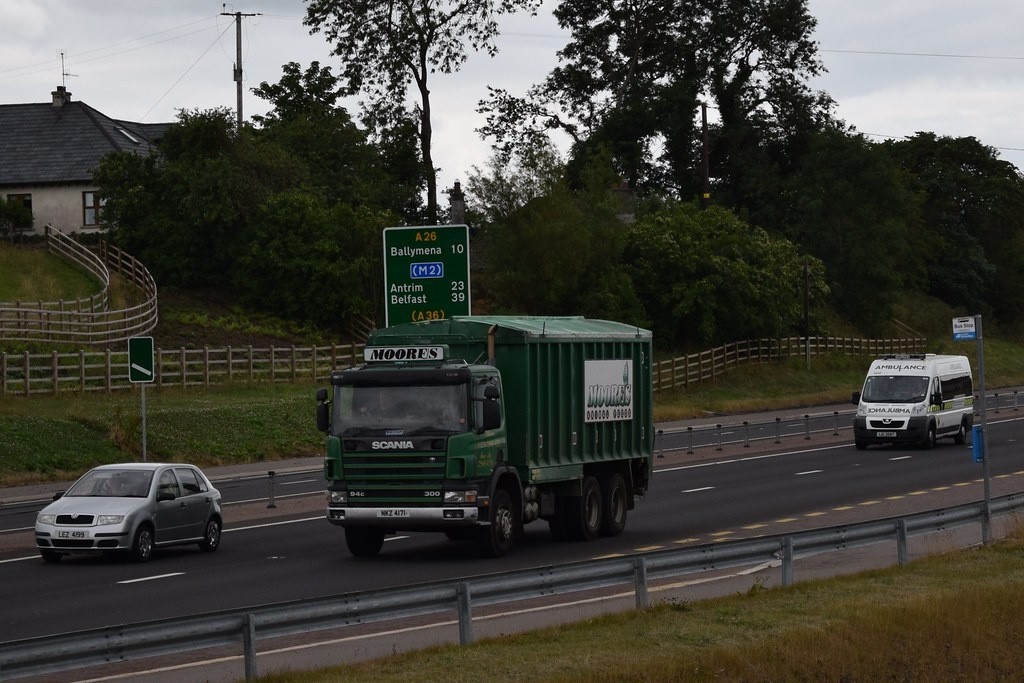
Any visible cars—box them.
[34,461,223,562]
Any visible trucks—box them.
[315,315,655,550]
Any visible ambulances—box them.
[853,350,972,448]
